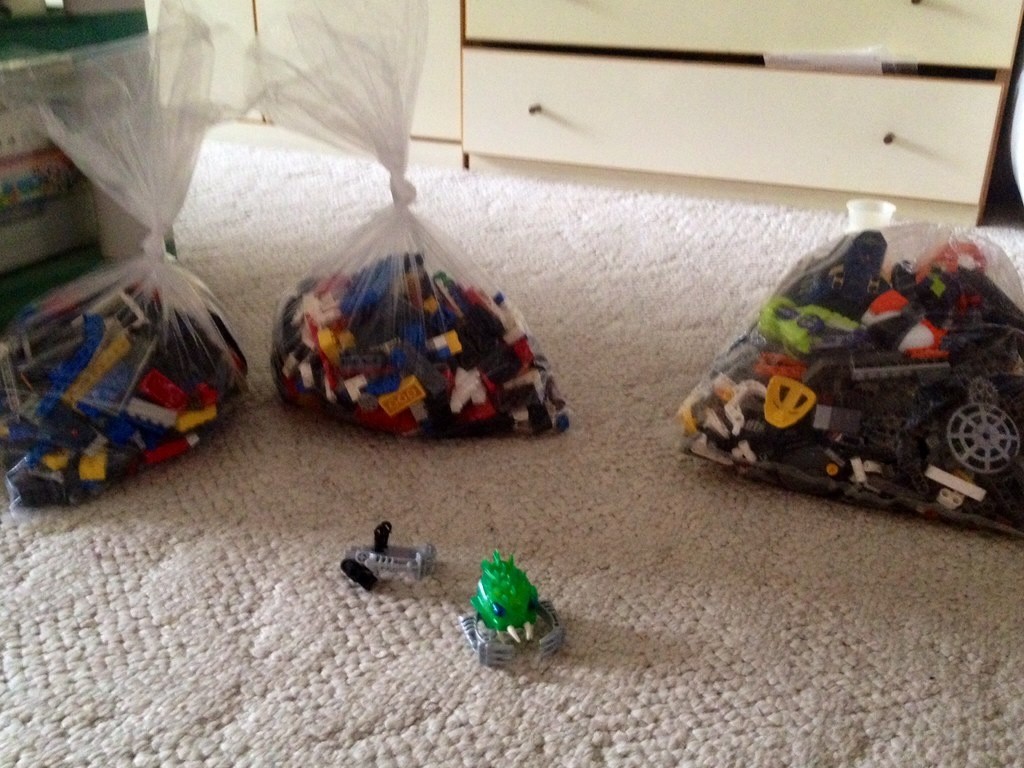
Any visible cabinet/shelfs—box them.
[459,0,1024,227]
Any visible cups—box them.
[847,198,897,233]
